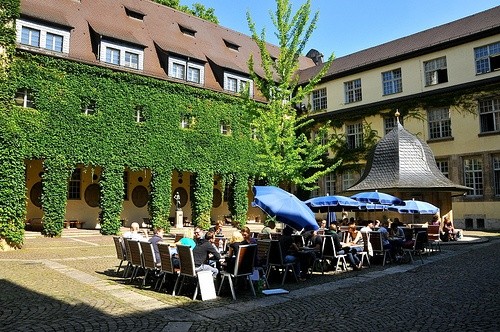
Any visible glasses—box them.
[194,230,201,234]
[219,226,222,227]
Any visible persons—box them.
[120,215,455,282]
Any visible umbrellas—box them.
[303,190,440,224]
[251,186,320,233]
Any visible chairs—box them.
[113,223,440,302]
